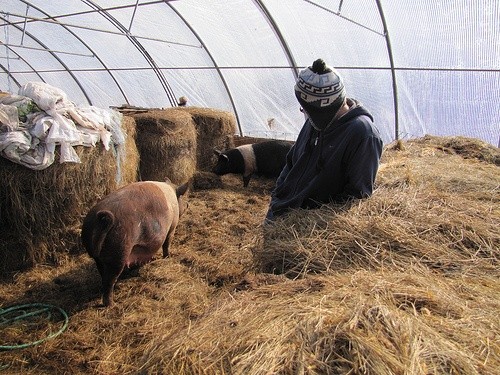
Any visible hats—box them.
[294,59,346,132]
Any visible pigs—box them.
[213,140,294,187]
[82,176,188,306]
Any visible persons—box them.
[265,59,383,280]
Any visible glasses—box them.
[299,106,306,113]
